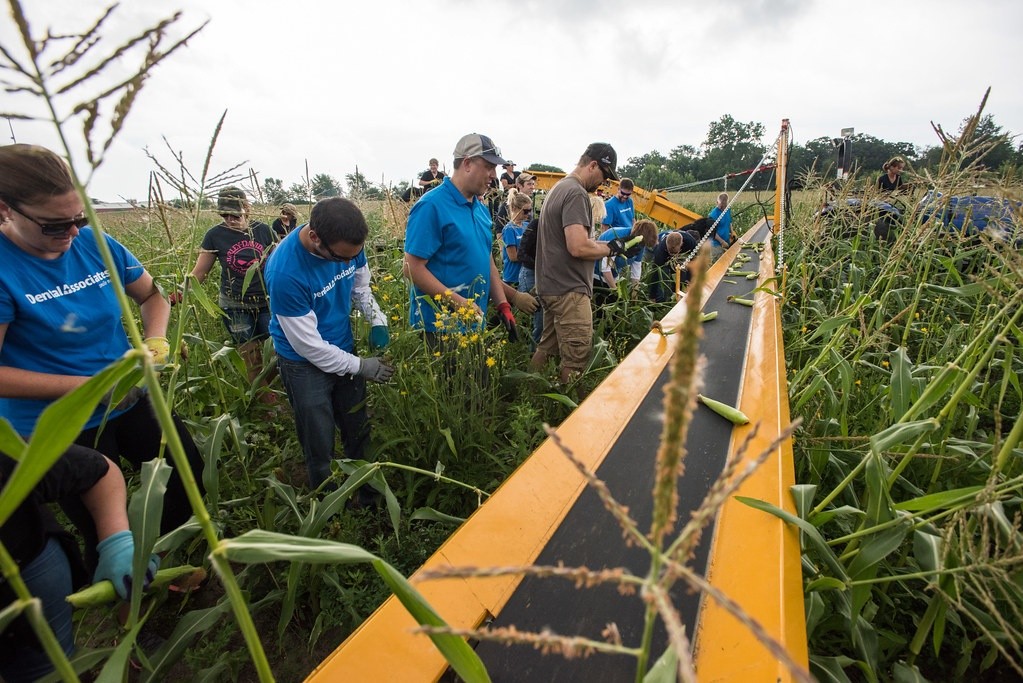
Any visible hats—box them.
[283,204,302,218]
[217,185,246,217]
[503,160,515,169]
[587,143,620,182]
[454,133,509,166]
[515,172,536,186]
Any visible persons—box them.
[0,132,736,683]
[877,156,908,194]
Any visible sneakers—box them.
[165,566,207,594]
[114,620,168,670]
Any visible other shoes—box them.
[328,496,374,521]
[344,472,385,494]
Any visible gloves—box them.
[613,286,622,296]
[368,325,389,352]
[649,298,655,307]
[494,302,518,343]
[510,290,540,315]
[357,356,393,384]
[607,236,641,258]
[141,336,170,383]
[99,380,140,410]
[167,288,183,306]
[630,289,638,306]
[90,531,161,600]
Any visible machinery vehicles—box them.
[821,185,1023,252]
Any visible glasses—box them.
[619,188,631,196]
[590,160,612,180]
[2,198,90,235]
[513,205,532,215]
[317,235,364,262]
[468,146,502,158]
[219,214,242,218]
